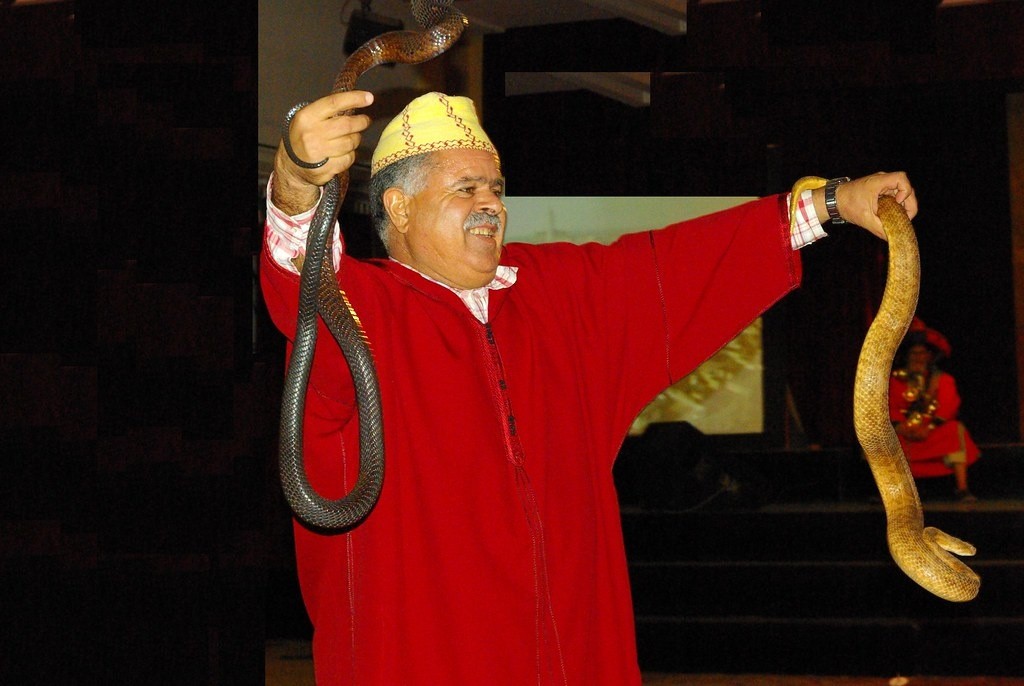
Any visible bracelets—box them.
[826,177,851,224]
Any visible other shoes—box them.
[954,488,979,506]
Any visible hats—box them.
[370,91,500,174]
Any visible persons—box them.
[259,90,919,686]
[888,318,979,504]
